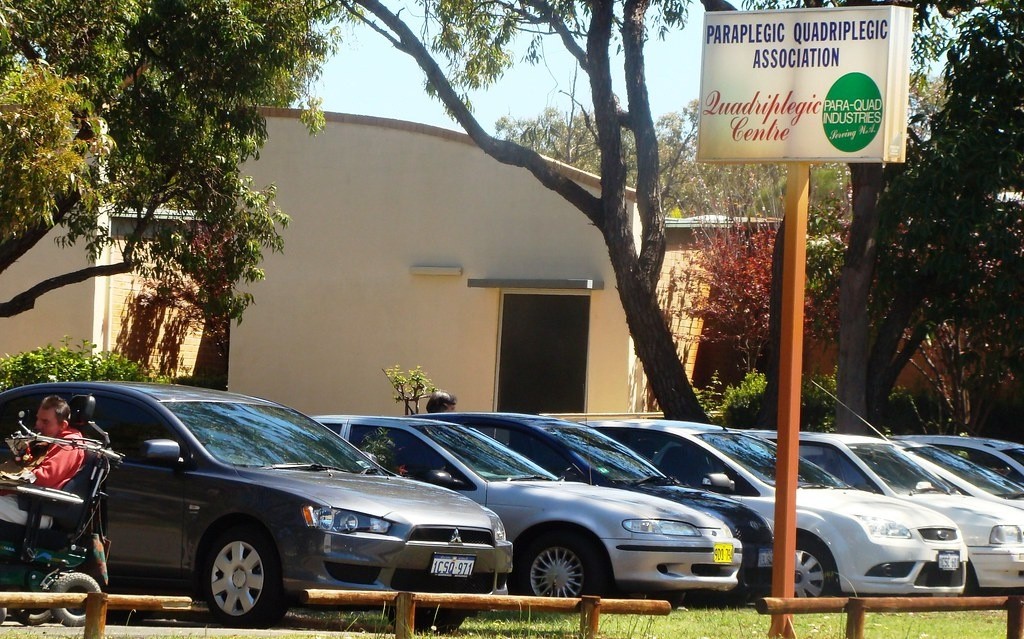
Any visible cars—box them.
[0,382,518,637]
[386,410,774,606]
[296,413,745,611]
[535,414,973,603]
[890,434,1024,502]
[645,430,1024,590]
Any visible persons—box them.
[0,395,85,529]
[426,390,457,413]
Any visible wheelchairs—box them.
[0,394,125,632]
